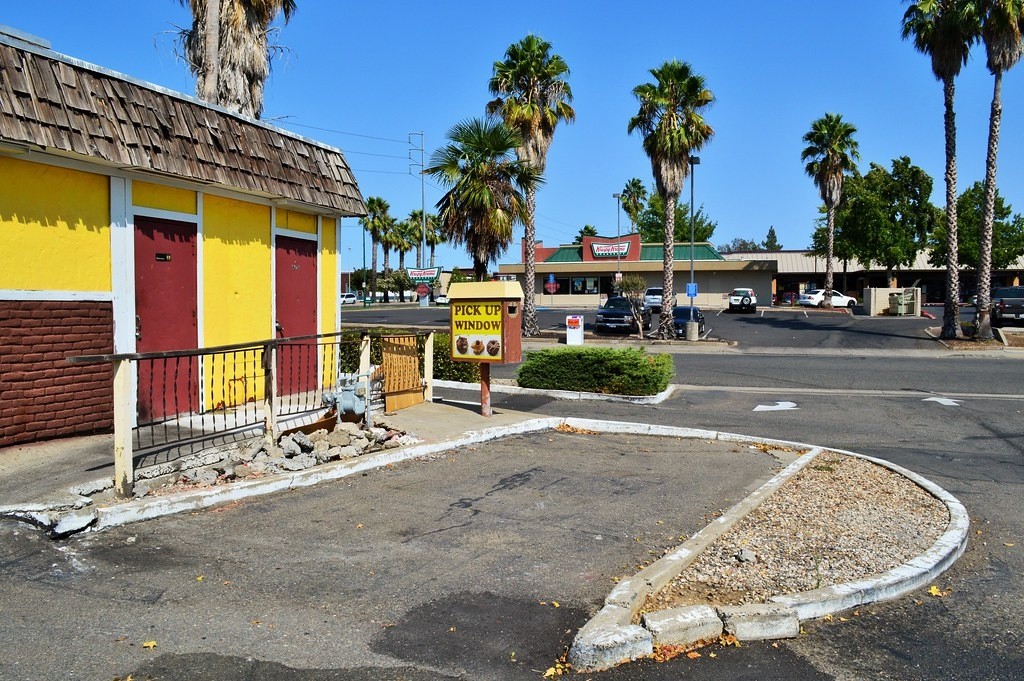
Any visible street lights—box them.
[613,192,621,296]
[687,154,700,323]
[346,247,352,293]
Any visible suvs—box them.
[644,286,678,310]
[728,287,757,314]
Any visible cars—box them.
[672,305,706,339]
[436,294,451,306]
[773,292,797,303]
[595,296,653,334]
[341,293,356,305]
[971,295,977,305]
[798,288,857,309]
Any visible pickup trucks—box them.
[990,287,1024,328]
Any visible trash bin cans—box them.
[889,295,911,316]
